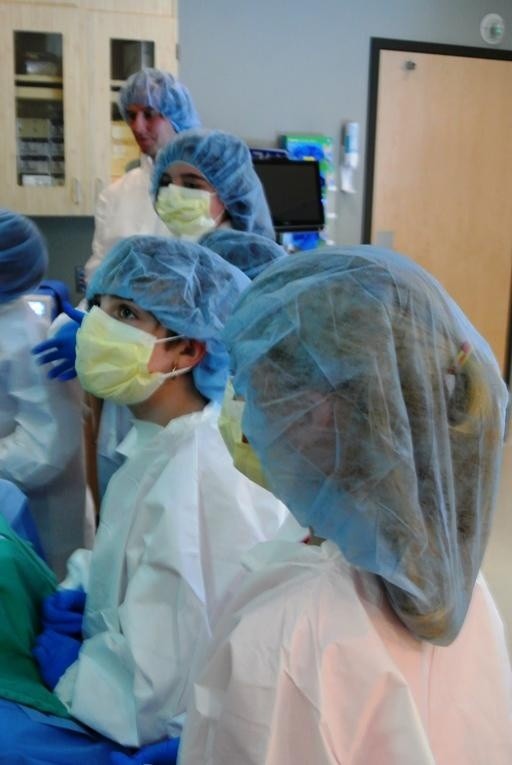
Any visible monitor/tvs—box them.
[252,158,327,232]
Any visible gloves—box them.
[31,301,85,383]
[32,590,86,693]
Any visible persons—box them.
[0,209,85,595]
[87,69,202,292]
[151,130,290,282]
[182,245,512,765]
[35,233,304,752]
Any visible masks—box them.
[75,305,192,406]
[217,375,272,494]
[154,184,226,245]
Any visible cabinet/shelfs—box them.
[0,1,182,217]
